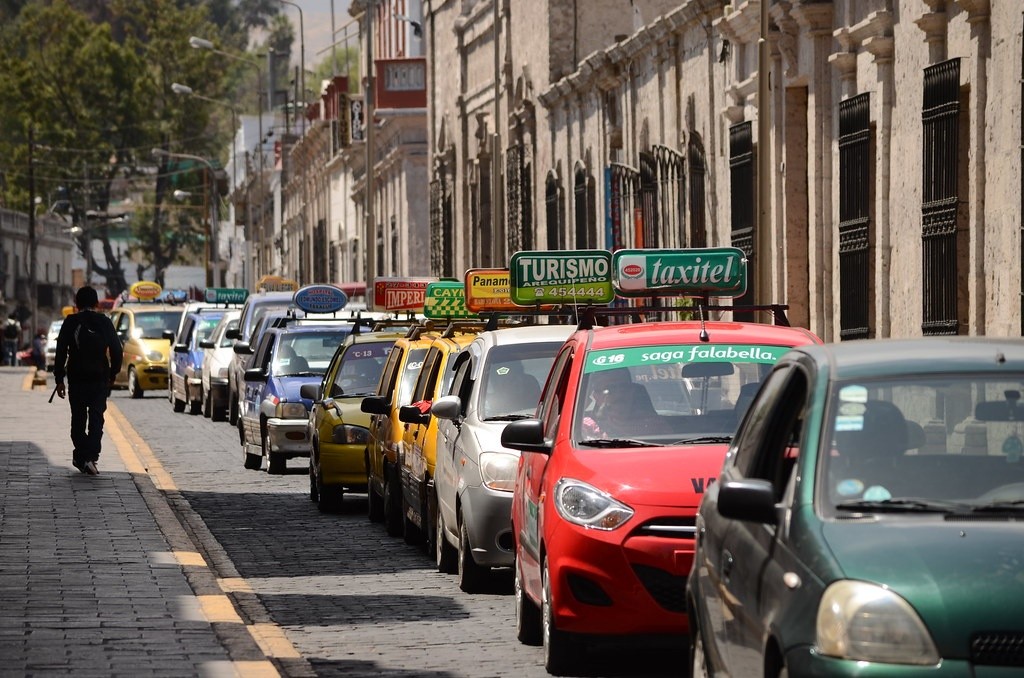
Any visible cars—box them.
[306,331,408,514]
[500,320,824,678]
[199,309,238,422]
[163,305,231,415]
[41,320,70,371]
[239,321,375,473]
[687,338,1024,678]
[360,319,511,523]
[432,324,606,595]
[104,303,183,399]
[399,322,482,558]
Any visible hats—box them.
[75,286,98,307]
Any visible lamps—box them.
[395,13,423,44]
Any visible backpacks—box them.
[5,319,17,338]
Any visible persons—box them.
[0,313,22,366]
[53,286,122,475]
[581,367,672,441]
[32,334,45,370]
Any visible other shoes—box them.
[84,460,98,476]
[72,454,86,473]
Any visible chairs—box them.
[492,372,542,417]
[833,394,910,471]
[273,344,310,373]
[721,380,763,435]
[630,382,676,437]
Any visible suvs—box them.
[234,307,318,415]
[225,288,297,426]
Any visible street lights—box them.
[190,36,268,274]
[170,84,242,287]
[151,148,220,288]
[31,225,83,343]
[174,189,222,289]
